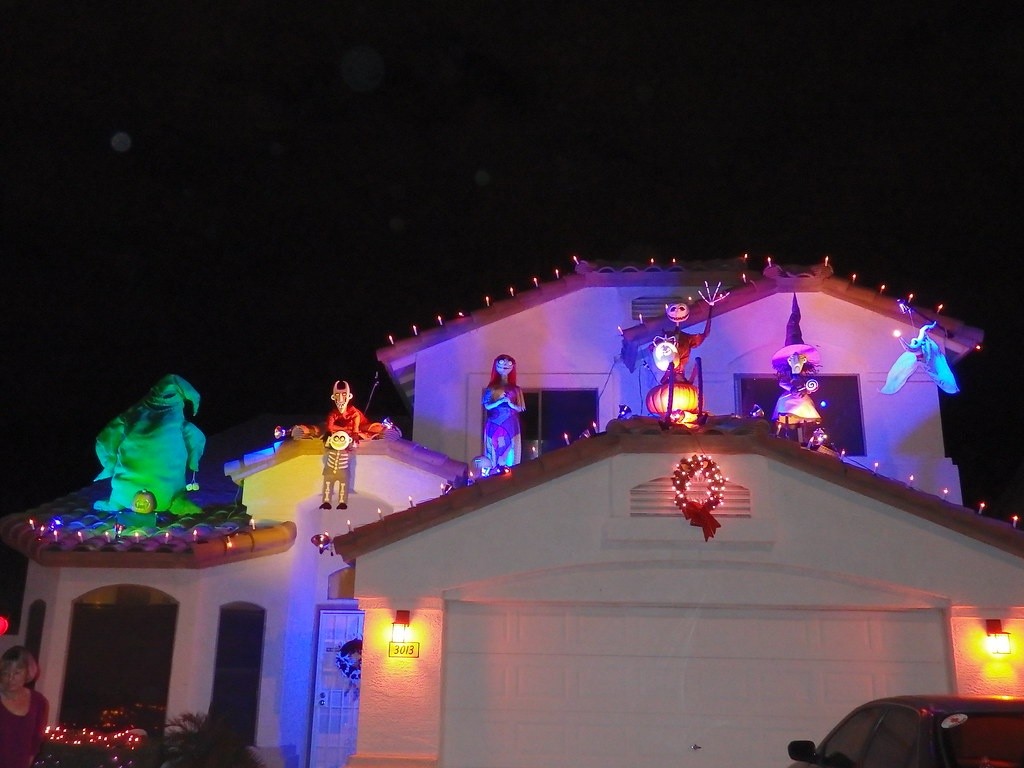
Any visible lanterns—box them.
[132,488,156,514]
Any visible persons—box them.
[482,353,527,476]
[318,431,354,510]
[771,346,823,445]
[291,379,384,496]
[0,645,51,768]
[646,280,723,427]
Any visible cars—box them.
[787,692,1024,768]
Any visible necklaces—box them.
[3,690,17,701]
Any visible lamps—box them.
[985,619,1011,654]
[391,610,411,642]
